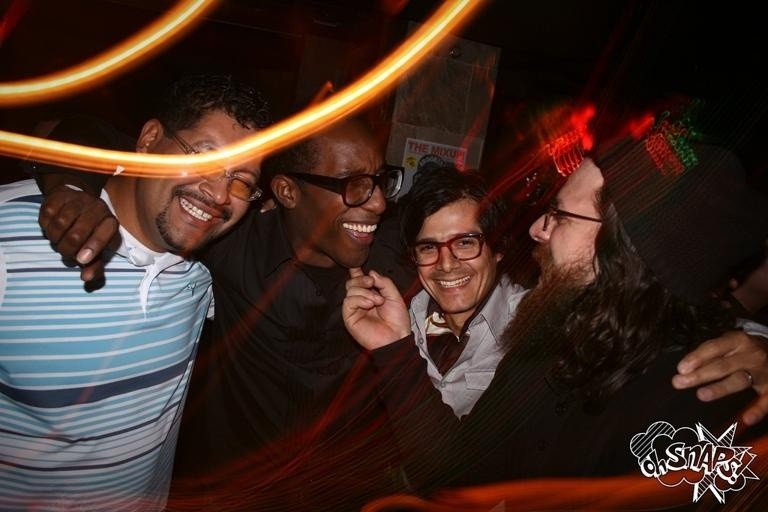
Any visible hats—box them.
[588,93,765,306]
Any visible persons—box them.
[0,69,276,510]
[397,159,534,420]
[27,99,418,512]
[670,252,768,424]
[339,126,737,505]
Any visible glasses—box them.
[279,164,406,207]
[150,115,263,202]
[544,200,606,231]
[408,233,485,267]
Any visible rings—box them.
[741,368,753,386]
